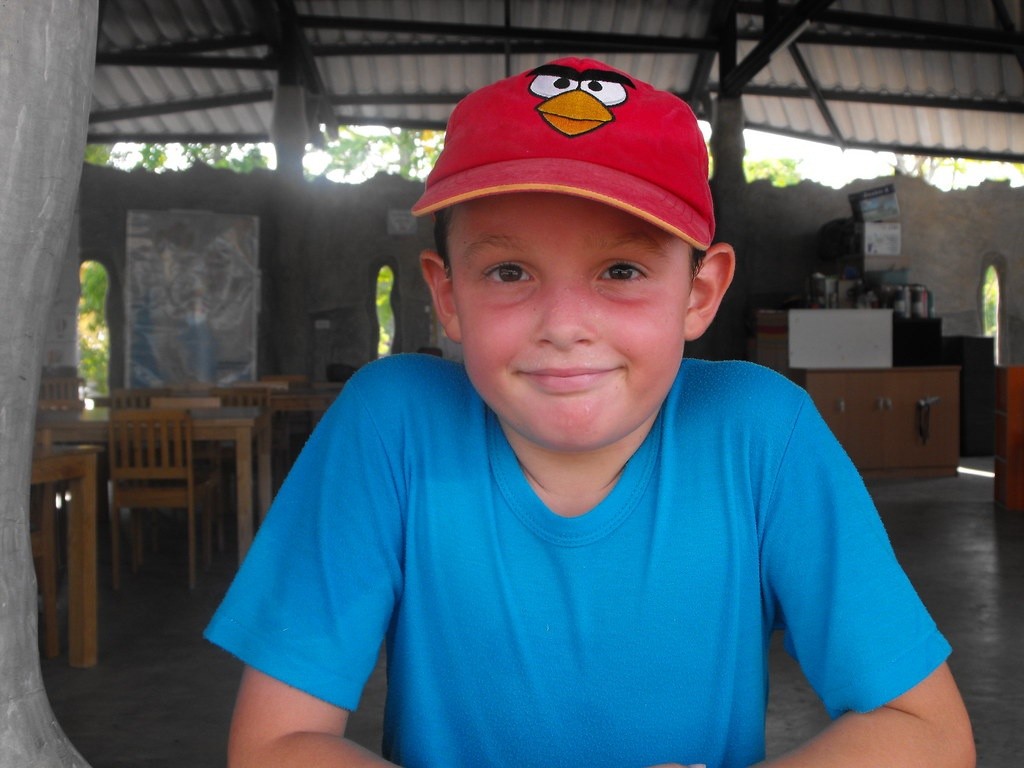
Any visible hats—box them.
[410,56,716,255]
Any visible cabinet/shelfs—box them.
[993,363,1024,513]
[804,363,964,483]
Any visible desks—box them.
[34,403,260,571]
[28,443,104,668]
[146,386,334,429]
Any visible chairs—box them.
[29,373,348,670]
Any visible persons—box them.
[221,52,978,768]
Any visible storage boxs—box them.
[787,306,896,369]
